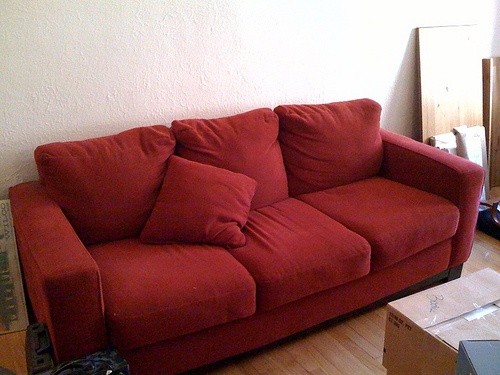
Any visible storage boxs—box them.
[382,267,500,375]
[454,340,500,375]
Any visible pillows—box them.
[141,154,257,247]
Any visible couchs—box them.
[9,98,487,375]
